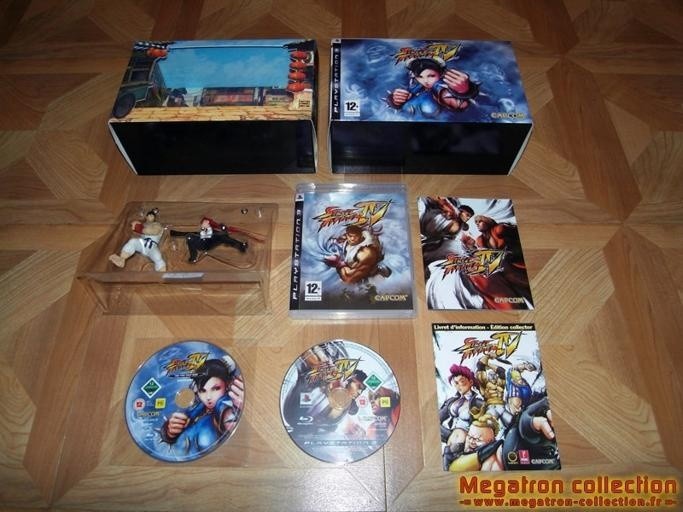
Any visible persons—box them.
[367,387,400,438]
[438,352,556,471]
[161,359,244,452]
[109,210,166,273]
[387,59,469,120]
[329,369,368,418]
[419,197,533,309]
[325,226,383,285]
[170,217,248,262]
